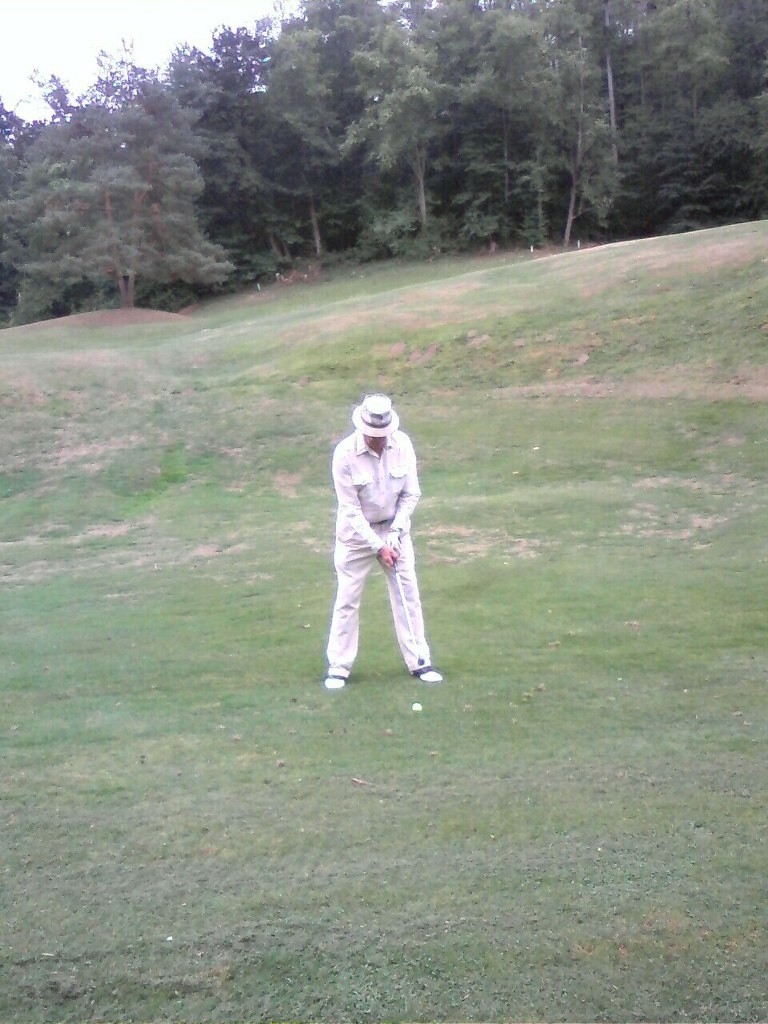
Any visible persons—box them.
[325,393,444,689]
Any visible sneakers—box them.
[413,667,442,683]
[325,674,347,690]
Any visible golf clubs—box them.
[392,558,425,666]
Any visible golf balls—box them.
[411,703,423,711]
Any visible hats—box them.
[352,394,400,437]
